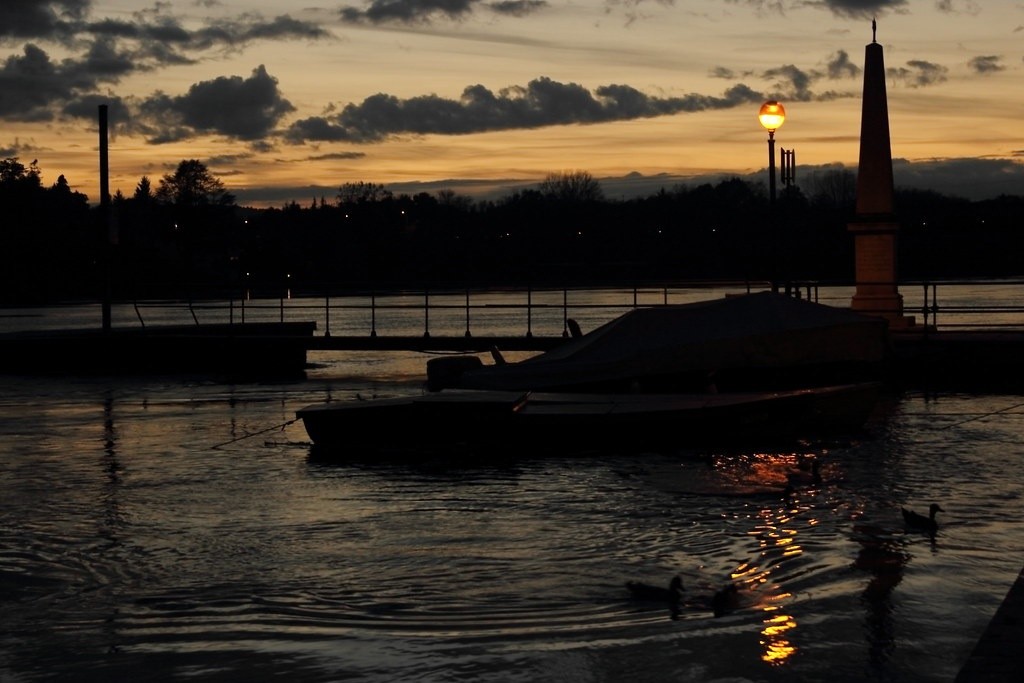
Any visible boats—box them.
[296,283,908,457]
[0,318,316,380]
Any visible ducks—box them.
[624,576,743,606]
[901,503,946,530]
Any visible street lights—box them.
[757,98,788,289]
